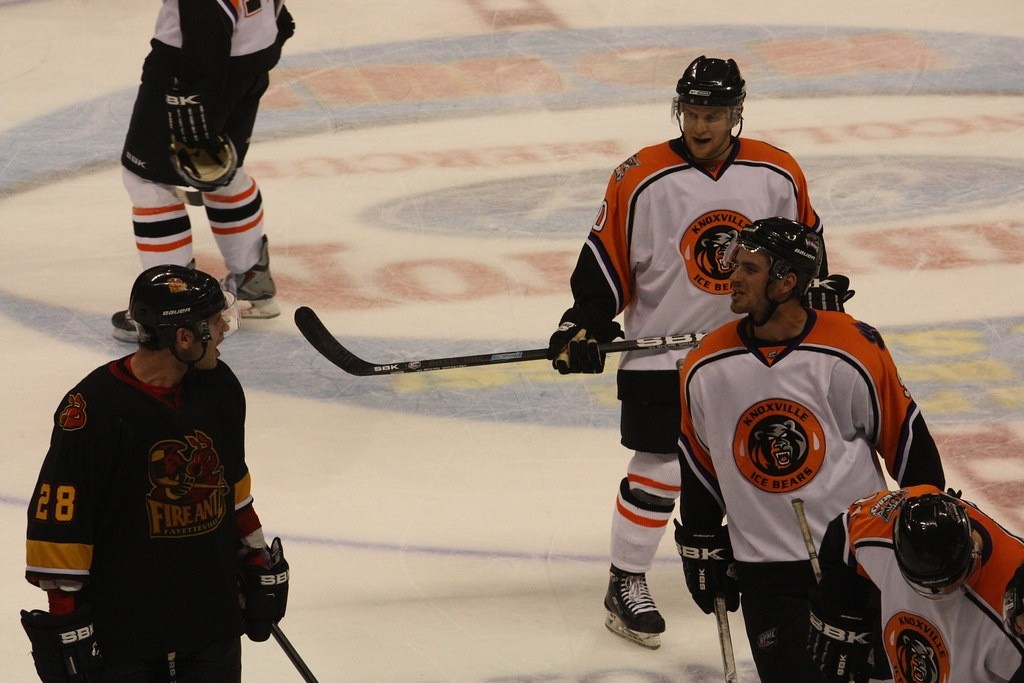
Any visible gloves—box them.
[546,307,626,376]
[672,518,740,615]
[238,535,289,643]
[18,607,104,683]
[162,85,228,148]
[806,273,855,313]
[806,598,874,683]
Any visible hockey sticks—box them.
[791,496,823,583]
[716,600,740,683]
[267,625,317,683]
[291,305,710,375]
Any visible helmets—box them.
[1005,560,1024,626]
[129,263,230,330]
[892,492,973,590]
[674,54,747,107]
[736,215,824,299]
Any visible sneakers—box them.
[219,232,281,319]
[603,562,666,651]
[110,258,197,343]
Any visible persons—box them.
[808,484,1024,683]
[675,217,945,683]
[19,266,290,683]
[112,0,295,343]
[547,56,855,649]
